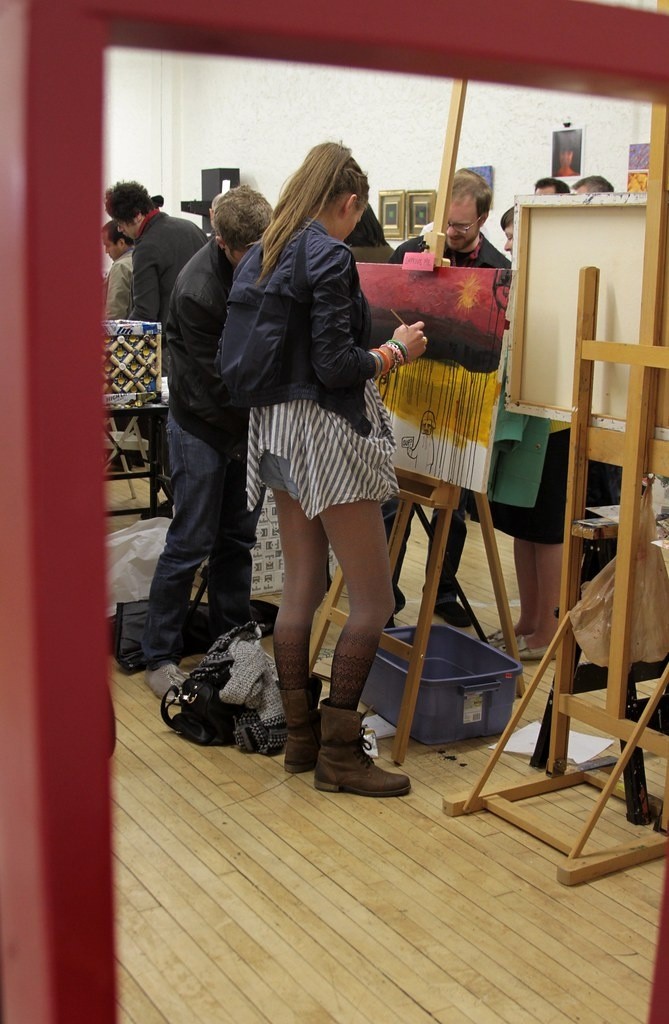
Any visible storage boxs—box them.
[104,318,162,405]
[358,621,524,746]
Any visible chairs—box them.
[105,411,149,500]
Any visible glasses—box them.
[447,214,483,234]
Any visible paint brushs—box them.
[391,309,408,329]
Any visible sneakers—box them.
[144,664,190,704]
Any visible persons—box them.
[103,181,208,503]
[215,142,427,798]
[470,177,624,665]
[205,193,225,240]
[139,184,274,704]
[380,168,514,629]
[326,201,395,592]
[552,137,580,177]
[101,220,145,471]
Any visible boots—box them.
[276,672,322,773]
[314,698,411,797]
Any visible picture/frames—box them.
[406,188,439,241]
[377,190,404,242]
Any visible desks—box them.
[101,401,171,519]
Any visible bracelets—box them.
[368,339,408,378]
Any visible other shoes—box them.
[487,629,557,660]
[436,601,472,627]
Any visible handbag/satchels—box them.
[569,475,669,666]
[160,679,246,747]
